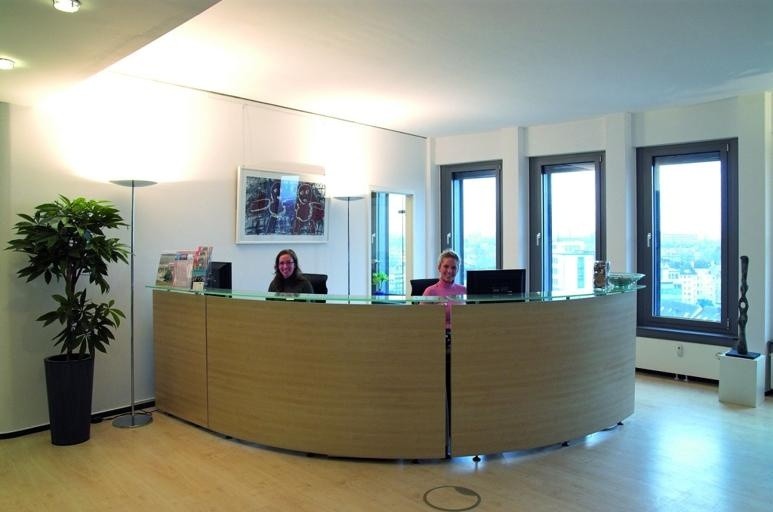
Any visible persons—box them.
[416,250,468,354]
[265,249,314,302]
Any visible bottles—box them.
[593,261,610,294]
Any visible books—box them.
[153,245,213,291]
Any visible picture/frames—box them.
[235,164,331,243]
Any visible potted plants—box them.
[372,272,389,295]
[3,194,136,446]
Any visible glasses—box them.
[277,261,295,265]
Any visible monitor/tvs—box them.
[206,261,233,298]
[466,268,528,304]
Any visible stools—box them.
[719,349,766,407]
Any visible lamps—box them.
[334,197,365,304]
[108,180,158,428]
